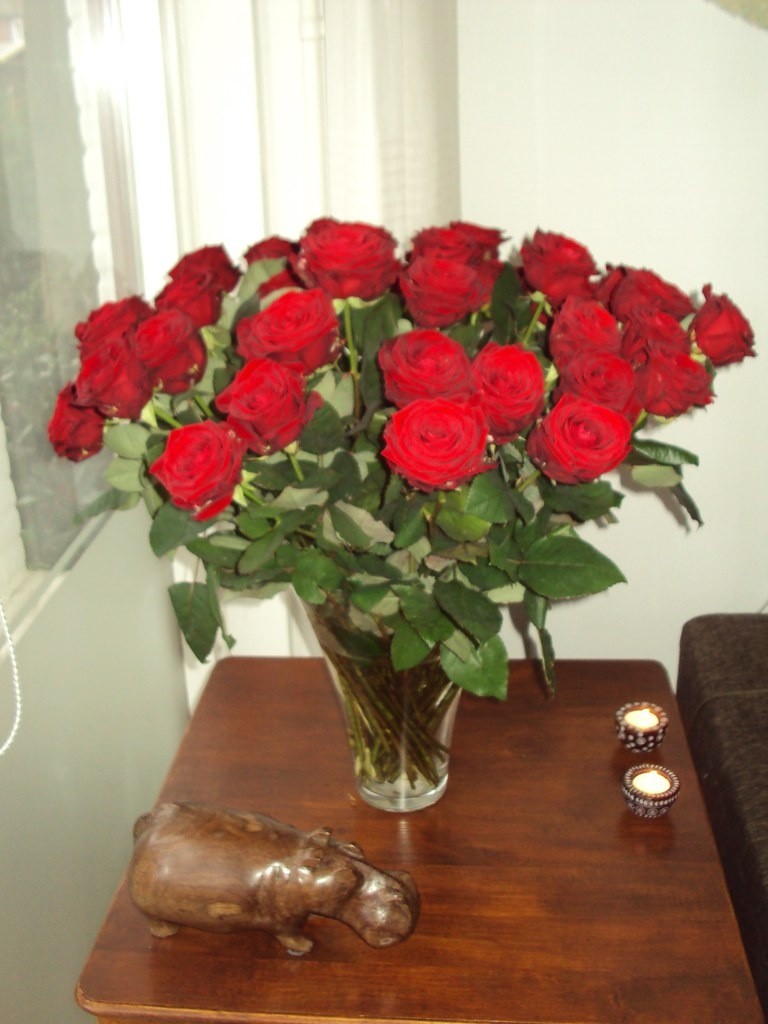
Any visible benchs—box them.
[675,612,767,1023]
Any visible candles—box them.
[632,770,671,794]
[625,706,659,728]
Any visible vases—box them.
[287,579,464,812]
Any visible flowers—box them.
[44,216,759,793]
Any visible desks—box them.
[73,658,765,1024]
